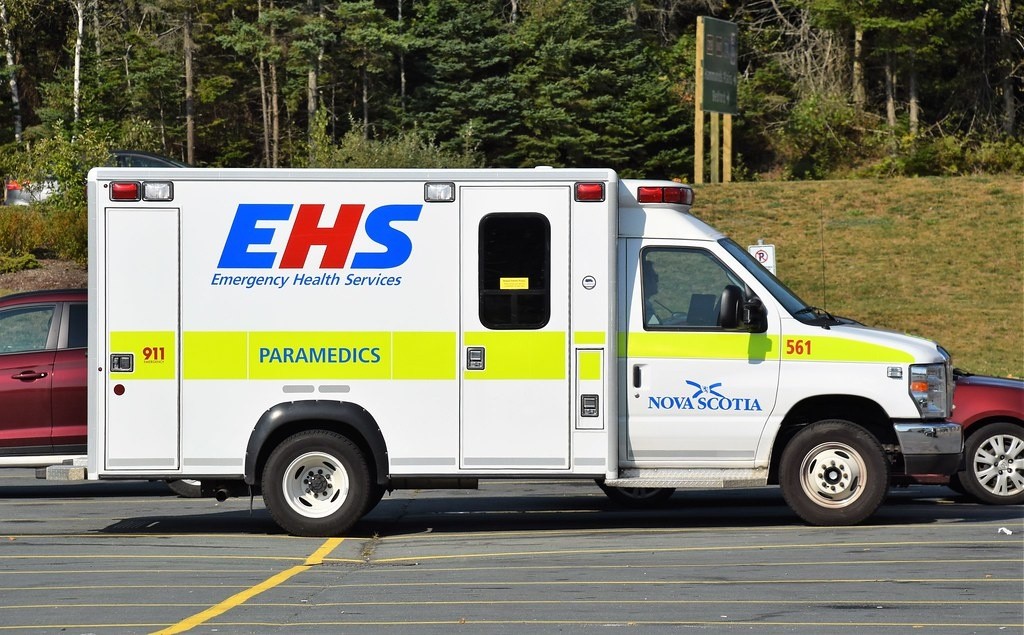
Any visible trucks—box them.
[88,167,966,538]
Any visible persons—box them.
[642,260,662,324]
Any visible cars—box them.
[0,290,88,469]
[895,365,1024,513]
[4,151,191,207]
